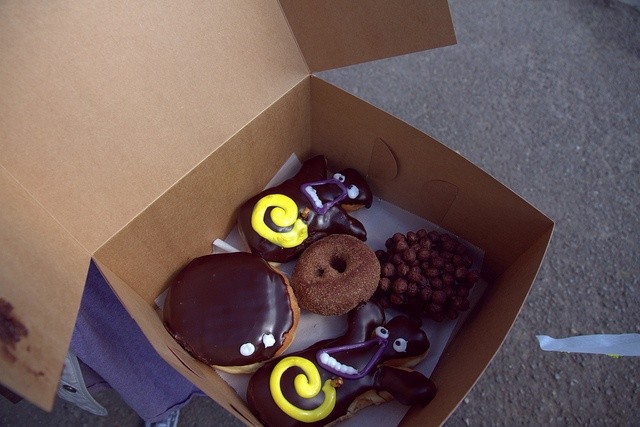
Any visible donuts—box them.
[293,233,381,317]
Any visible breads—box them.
[239,150,370,256]
[163,251,298,376]
[246,303,436,424]
[374,228,481,324]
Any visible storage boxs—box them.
[1,1,556,427]
